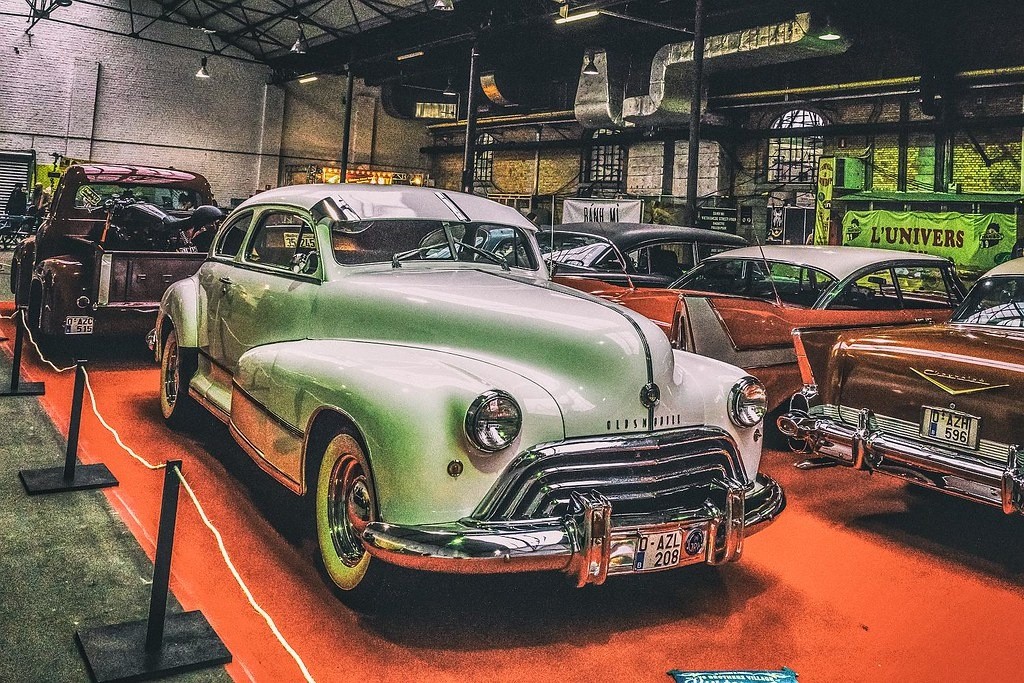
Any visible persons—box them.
[26,182,51,237]
[525,212,540,224]
[4,182,27,233]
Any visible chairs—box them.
[0,214,36,250]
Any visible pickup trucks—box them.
[9,162,226,351]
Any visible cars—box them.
[777,256,1023,515]
[146,183,786,604]
[552,243,968,450]
[538,222,749,292]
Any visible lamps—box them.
[289,29,308,55]
[195,57,210,77]
[817,15,841,43]
[553,9,599,25]
[434,0,455,12]
